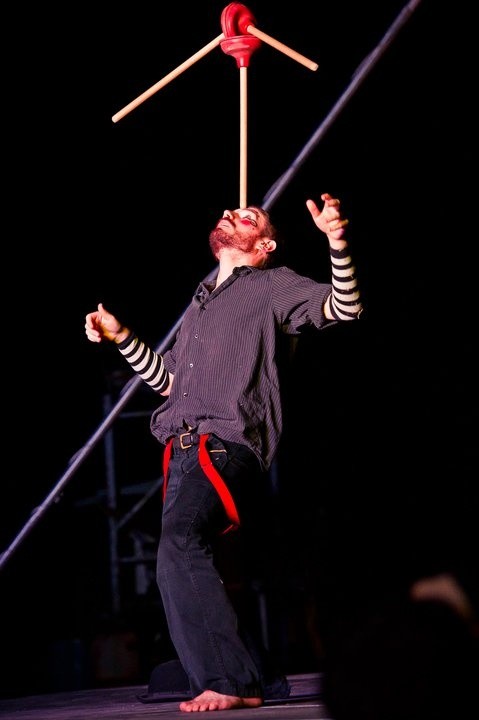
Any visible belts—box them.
[173,431,200,450]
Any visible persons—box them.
[83,192,361,712]
